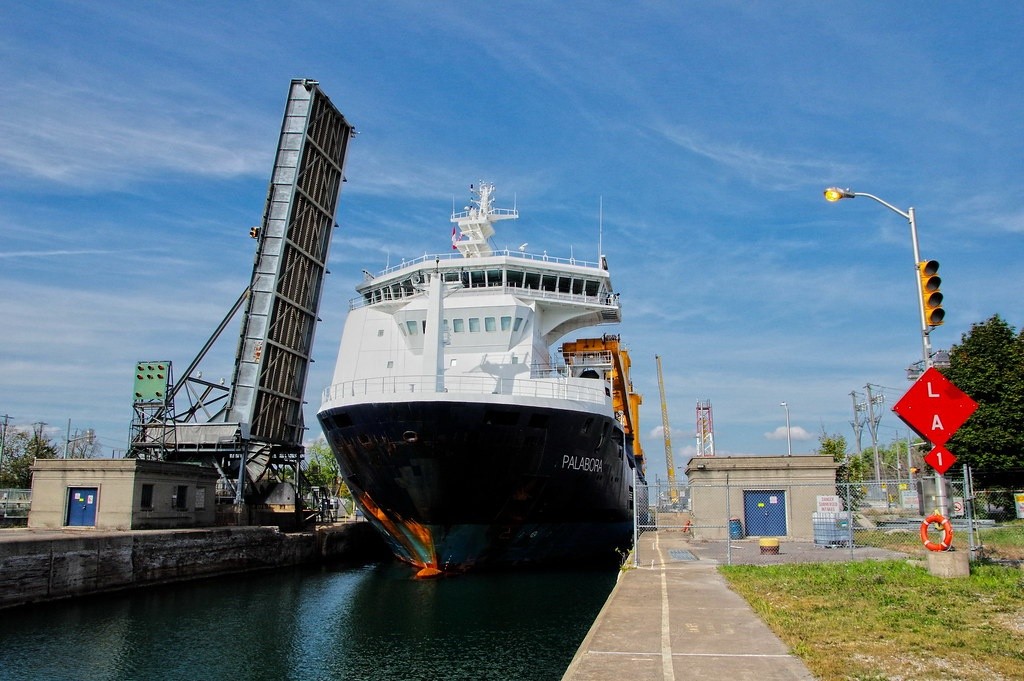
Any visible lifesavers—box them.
[919,515,954,551]
[683,520,690,533]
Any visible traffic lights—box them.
[920,260,945,327]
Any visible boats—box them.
[316,180,649,578]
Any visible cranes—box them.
[655,354,678,503]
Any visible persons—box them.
[606,293,620,303]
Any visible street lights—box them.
[780,402,791,455]
[824,187,953,551]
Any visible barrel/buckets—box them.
[729,521,743,540]
